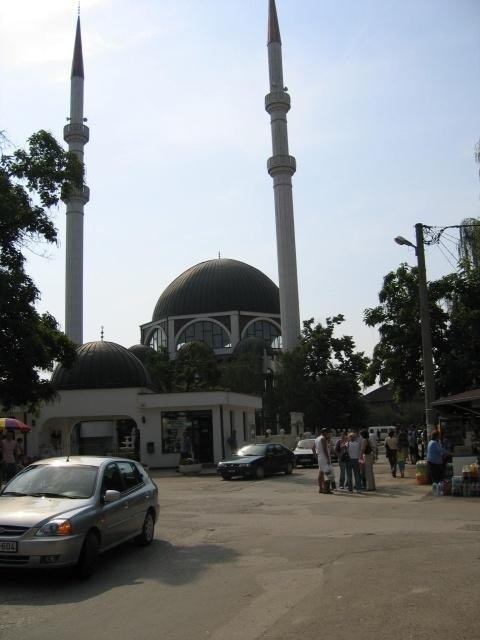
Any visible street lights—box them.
[394,224,437,442]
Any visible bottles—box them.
[433,463,480,497]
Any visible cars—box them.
[217,437,342,480]
[0,455,160,579]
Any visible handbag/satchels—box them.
[341,452,351,463]
[365,447,376,464]
[385,446,392,457]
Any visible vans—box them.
[368,426,396,440]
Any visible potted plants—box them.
[178,457,202,476]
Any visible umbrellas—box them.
[0,418,31,431]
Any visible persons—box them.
[262,432,272,443]
[15,438,23,464]
[0,431,19,478]
[427,431,454,483]
[296,424,427,493]
